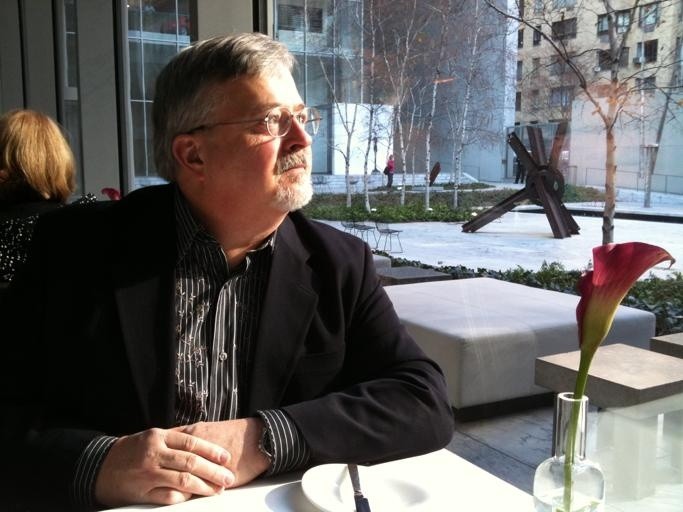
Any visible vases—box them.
[533,242,676,512]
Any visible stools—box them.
[649,333,683,440]
[535,343,683,501]
[376,266,453,286]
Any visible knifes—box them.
[347,460,371,511]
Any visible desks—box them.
[382,277,655,410]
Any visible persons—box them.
[513,156,526,183]
[2,33,455,511]
[1,108,95,293]
[387,154,395,187]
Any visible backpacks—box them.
[384,167,389,175]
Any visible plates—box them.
[301,463,428,511]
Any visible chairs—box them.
[341,214,404,252]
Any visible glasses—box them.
[185,107,321,137]
